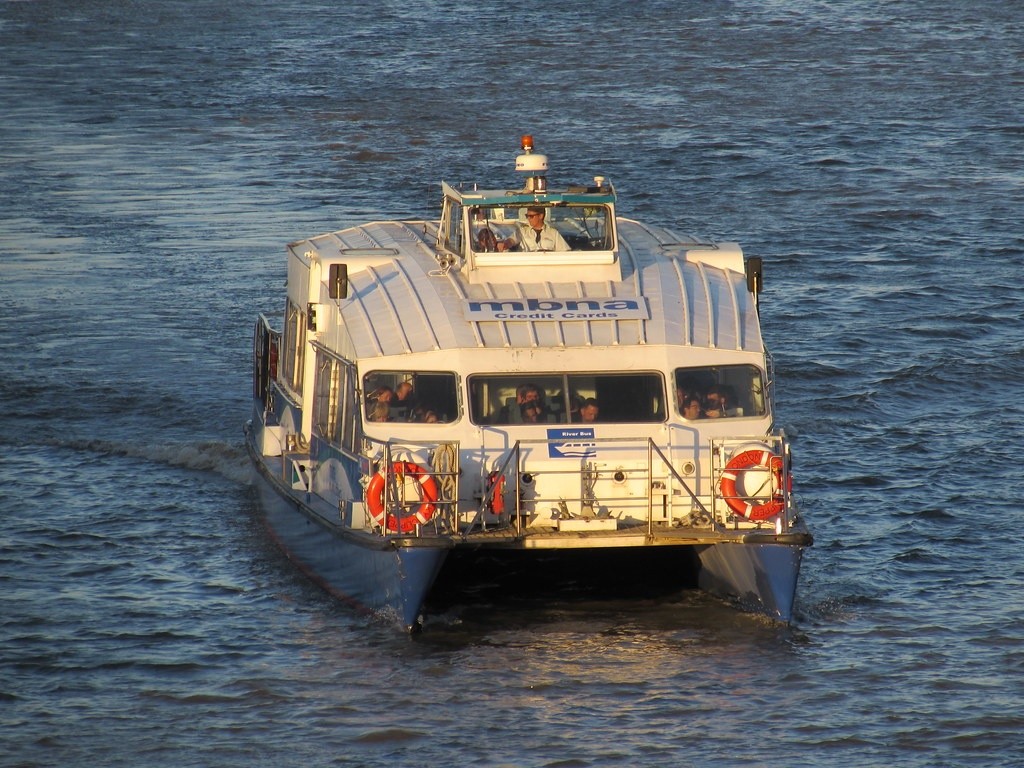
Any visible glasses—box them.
[525,214,539,219]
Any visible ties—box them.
[534,229,542,243]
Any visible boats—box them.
[243,133,817,641]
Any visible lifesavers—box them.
[366,460,438,534]
[721,449,793,522]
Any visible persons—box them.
[471,208,486,220]
[477,228,512,252]
[678,384,724,420]
[496,384,545,424]
[497,206,571,252]
[372,382,437,423]
[571,398,600,422]
